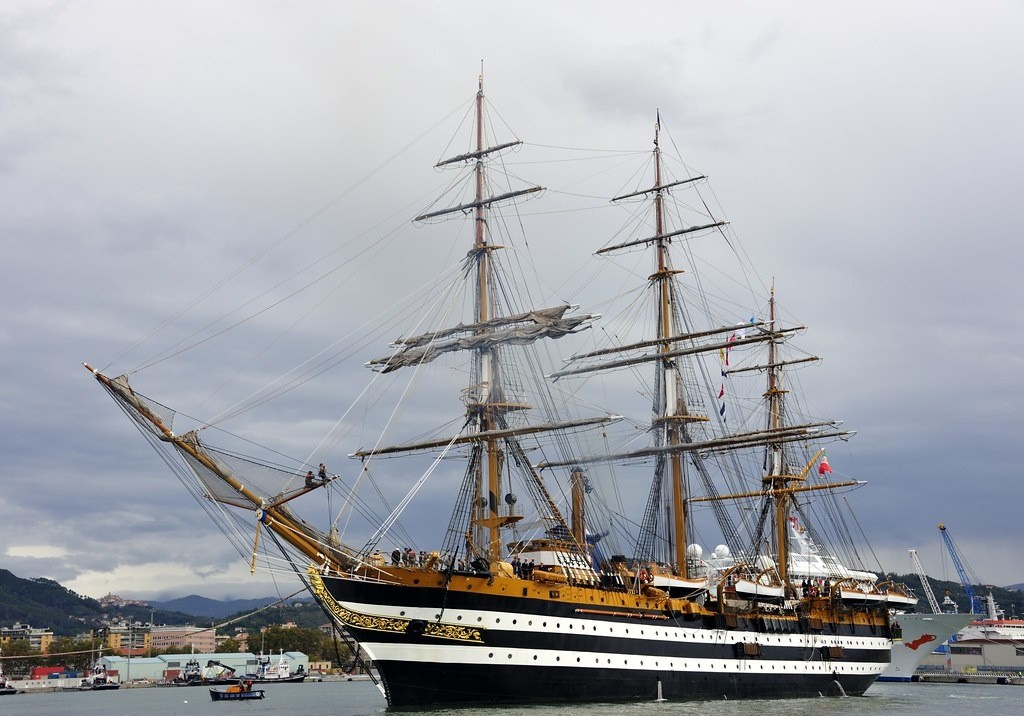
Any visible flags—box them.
[718,315,755,415]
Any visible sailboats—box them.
[80,48,923,716]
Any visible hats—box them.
[541,562,543,563]
[517,557,520,558]
[531,558,535,560]
[514,557,517,559]
[525,558,527,560]
[420,550,423,552]
[375,549,380,550]
[396,548,399,550]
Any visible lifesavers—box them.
[639,569,655,583]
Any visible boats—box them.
[687,542,985,682]
[0,686,18,695]
[76,686,92,691]
[178,656,304,683]
[208,687,266,702]
[93,684,121,690]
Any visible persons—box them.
[802,577,831,597]
[374,548,427,566]
[891,621,900,631]
[445,555,452,569]
[511,557,545,581]
[239,676,253,692]
[305,471,315,488]
[318,464,328,479]
[457,558,465,570]
